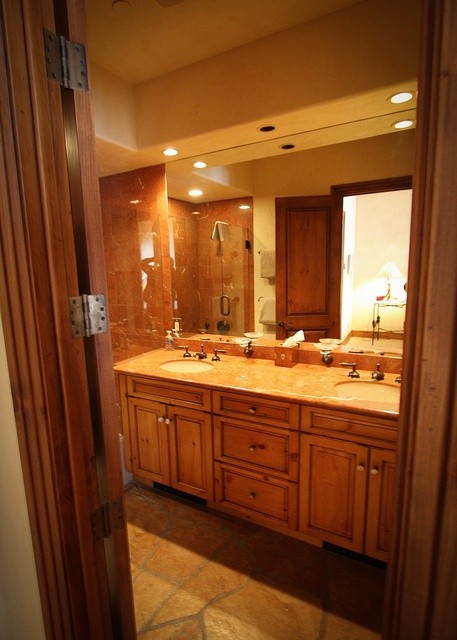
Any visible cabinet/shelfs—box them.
[212,390,299,538]
[300,404,398,565]
[116,372,214,513]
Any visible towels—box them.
[211,222,231,242]
[259,298,276,327]
[260,248,276,279]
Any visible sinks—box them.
[332,379,400,404]
[158,360,214,373]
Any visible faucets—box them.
[218,337,223,342]
[372,363,384,380]
[193,344,208,360]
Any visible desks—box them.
[372,301,405,343]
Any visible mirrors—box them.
[172,109,416,367]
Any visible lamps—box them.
[374,262,403,301]
